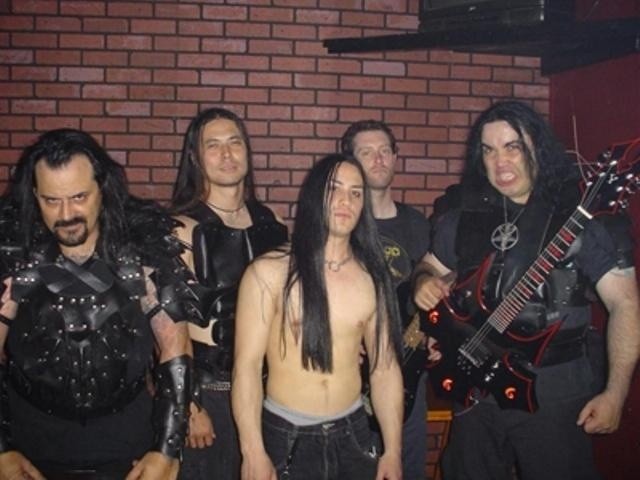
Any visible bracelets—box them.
[412,270,434,281]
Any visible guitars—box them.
[360,276,428,432]
[418,139,640,412]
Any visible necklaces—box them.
[323,254,353,271]
[490,194,528,252]
[206,201,245,213]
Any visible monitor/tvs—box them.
[418,0,576,34]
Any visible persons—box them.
[341,119,443,480]
[0,127,198,480]
[409,99,640,480]
[229,153,407,479]
[162,107,294,479]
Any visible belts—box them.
[542,341,583,367]
[200,379,231,391]
